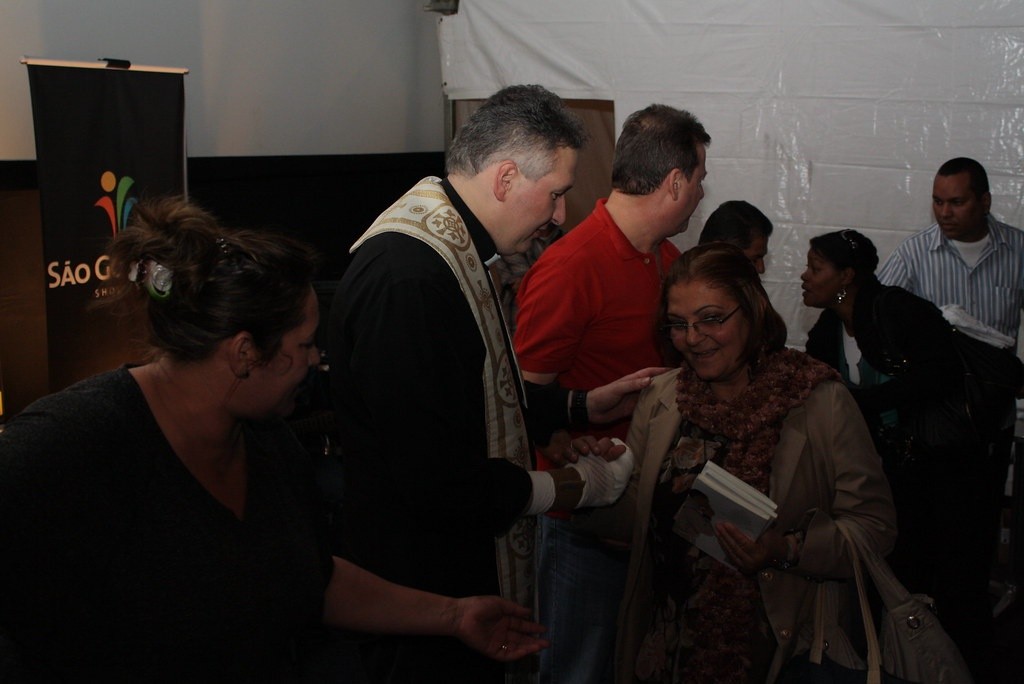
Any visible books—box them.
[674,459,777,572]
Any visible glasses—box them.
[659,302,743,340]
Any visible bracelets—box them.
[572,388,589,432]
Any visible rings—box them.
[501,644,507,651]
[570,439,574,447]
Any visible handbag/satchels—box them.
[823,519,973,684]
[773,520,909,684]
[870,285,996,490]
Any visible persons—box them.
[0,197,550,684]
[490,218,570,332]
[700,201,771,277]
[802,230,1024,619]
[899,157,1024,586]
[554,240,898,684]
[514,106,709,684]
[324,82,680,684]
[674,489,715,544]
